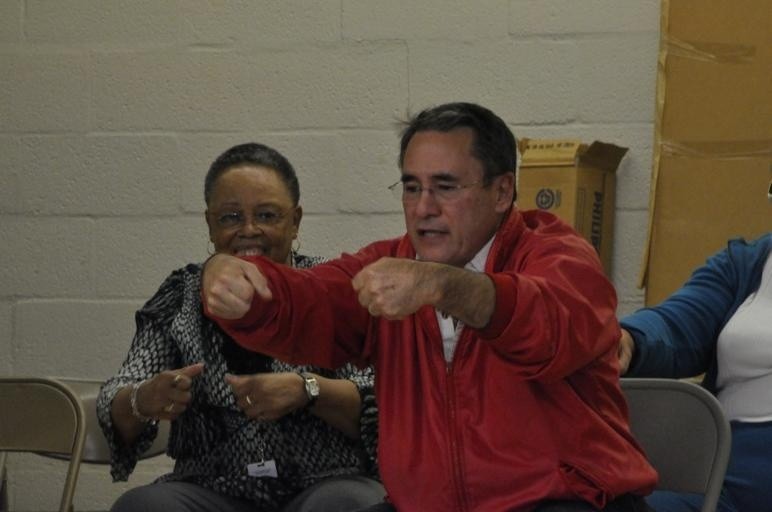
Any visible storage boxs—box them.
[511,136,630,280]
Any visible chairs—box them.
[616,379,732,510]
[0,376,88,510]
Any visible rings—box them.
[245,396,252,405]
[172,374,181,385]
[168,403,174,413]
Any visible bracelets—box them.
[129,379,156,426]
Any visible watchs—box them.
[298,372,321,408]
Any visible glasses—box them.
[389,179,482,200]
[212,210,293,225]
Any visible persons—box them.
[201,102,660,512]
[96,143,387,511]
[618,183,772,511]
